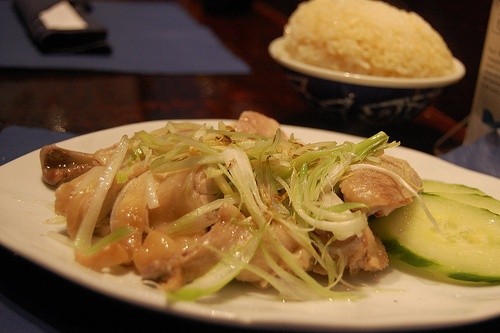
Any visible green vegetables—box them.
[73,120,402,300]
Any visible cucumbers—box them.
[422,179,500,214]
[370,190,500,286]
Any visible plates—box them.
[16,1,110,51]
[0,119,500,331]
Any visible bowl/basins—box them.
[269,36,466,122]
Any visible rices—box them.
[281,0,456,78]
